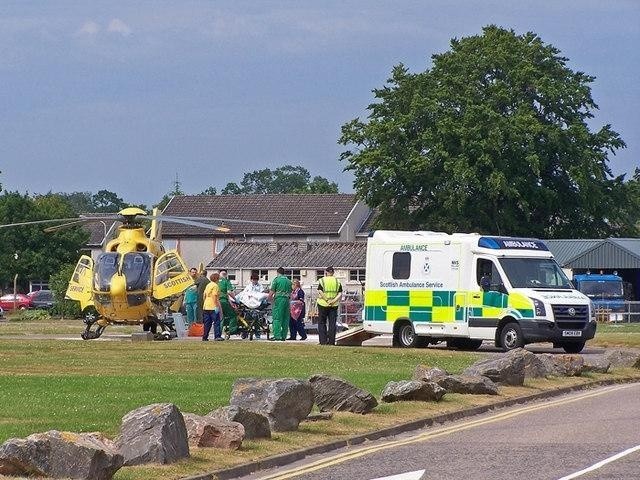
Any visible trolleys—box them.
[223,303,271,340]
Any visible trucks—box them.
[361,228,597,353]
[574,272,626,322]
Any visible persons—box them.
[217,269,241,335]
[287,278,307,340]
[202,273,225,341]
[246,272,267,339]
[195,270,210,325]
[316,266,342,346]
[268,267,292,341]
[184,268,199,330]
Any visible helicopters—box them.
[0,207,309,341]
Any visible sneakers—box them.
[203,330,307,341]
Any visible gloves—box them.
[262,300,267,304]
[290,301,297,304]
[215,307,219,314]
[235,298,240,304]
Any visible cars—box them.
[0,291,58,312]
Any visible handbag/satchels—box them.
[188,323,204,336]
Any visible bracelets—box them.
[326,299,329,303]
